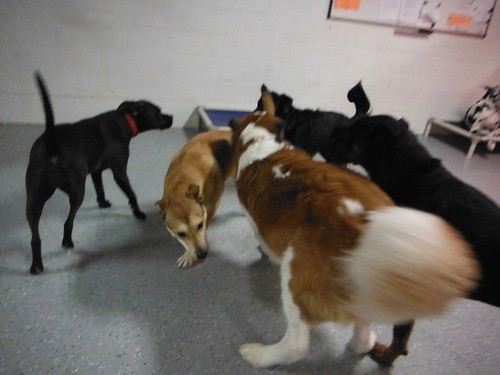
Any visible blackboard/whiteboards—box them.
[327,0,496,39]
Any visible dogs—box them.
[155,129,236,268]
[24,71,173,276]
[254,83,499,366]
[228,116,481,368]
